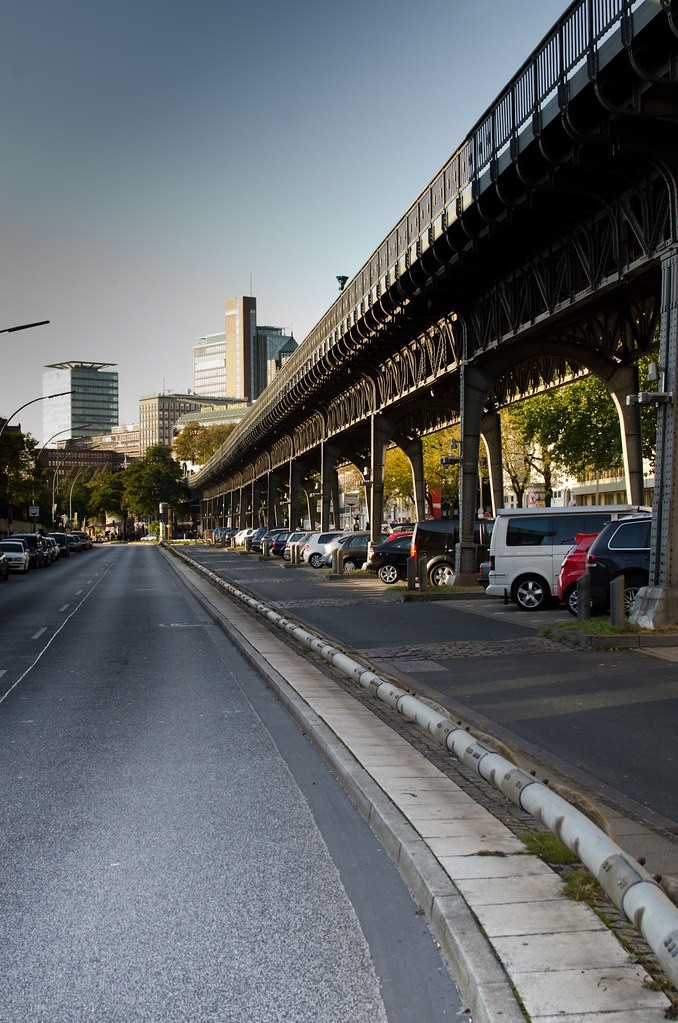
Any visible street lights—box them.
[53,444,100,532]
[0,391,76,438]
[33,424,92,532]
[69,465,95,531]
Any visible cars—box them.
[557,513,652,618]
[0,542,30,574]
[140,533,157,541]
[2,531,93,569]
[213,518,495,588]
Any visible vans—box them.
[485,504,653,609]
